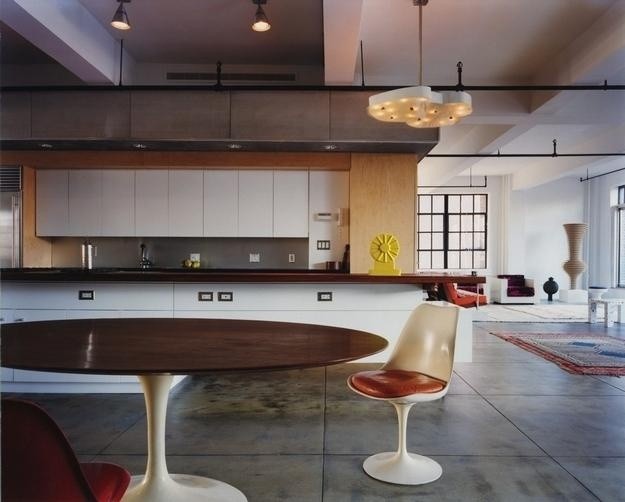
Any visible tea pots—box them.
[82,240,98,269]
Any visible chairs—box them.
[347,304,460,485]
[0,397,131,501]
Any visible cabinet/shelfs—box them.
[238,170,309,236]
[34,167,103,236]
[169,169,239,236]
[309,170,349,240]
[101,169,168,238]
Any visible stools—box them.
[588,299,625,328]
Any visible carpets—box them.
[491,332,624,377]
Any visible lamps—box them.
[110,2,133,31]
[250,1,272,32]
[367,0,473,130]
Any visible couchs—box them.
[490,275,541,305]
[444,282,486,307]
